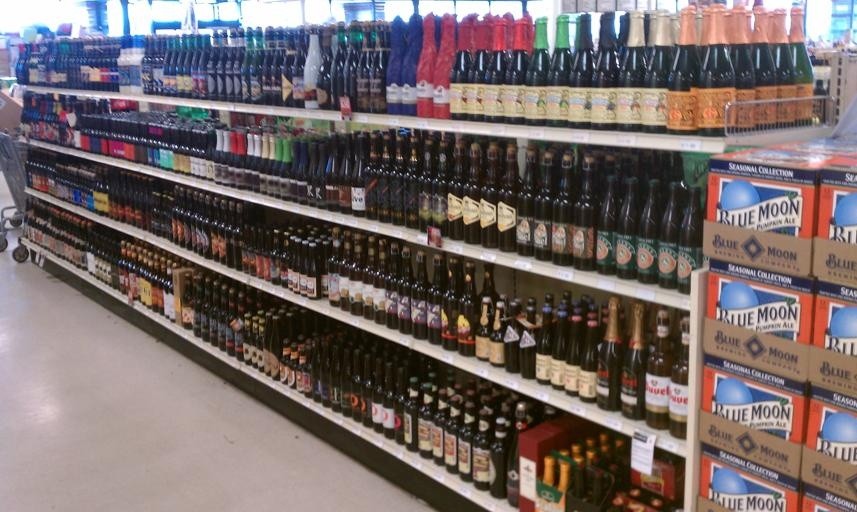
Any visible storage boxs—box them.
[802,282,856,411]
[811,147,856,302]
[0,87,24,137]
[702,139,857,293]
[801,483,856,511]
[798,386,855,512]
[698,443,800,511]
[698,353,811,493]
[702,257,815,401]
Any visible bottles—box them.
[15,1,815,510]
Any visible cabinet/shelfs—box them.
[18,83,840,512]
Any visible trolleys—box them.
[0,131,29,263]
